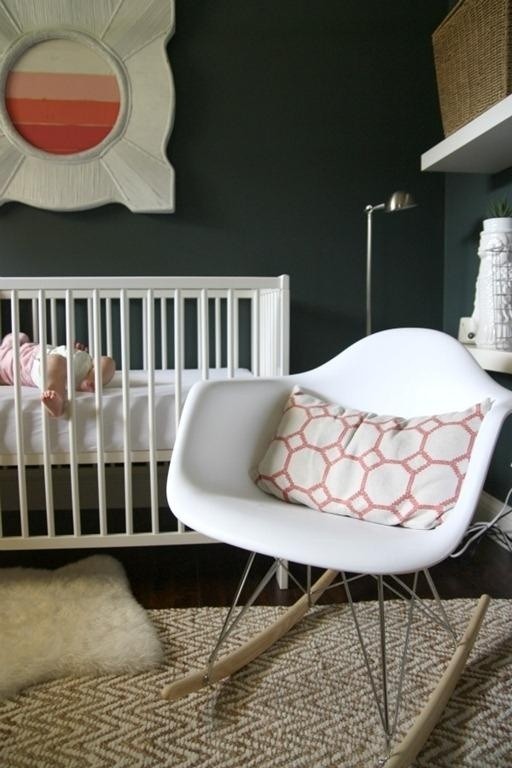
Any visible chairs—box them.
[163,326,511,767]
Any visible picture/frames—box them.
[0,0,175,216]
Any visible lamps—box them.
[364,190,418,337]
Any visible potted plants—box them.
[477,194,511,350]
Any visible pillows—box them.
[255,383,492,528]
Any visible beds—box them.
[1,270,292,591]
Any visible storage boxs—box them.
[432,0,512,140]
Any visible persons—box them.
[0,332,116,417]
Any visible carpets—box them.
[3,599,509,766]
[1,554,166,697]
